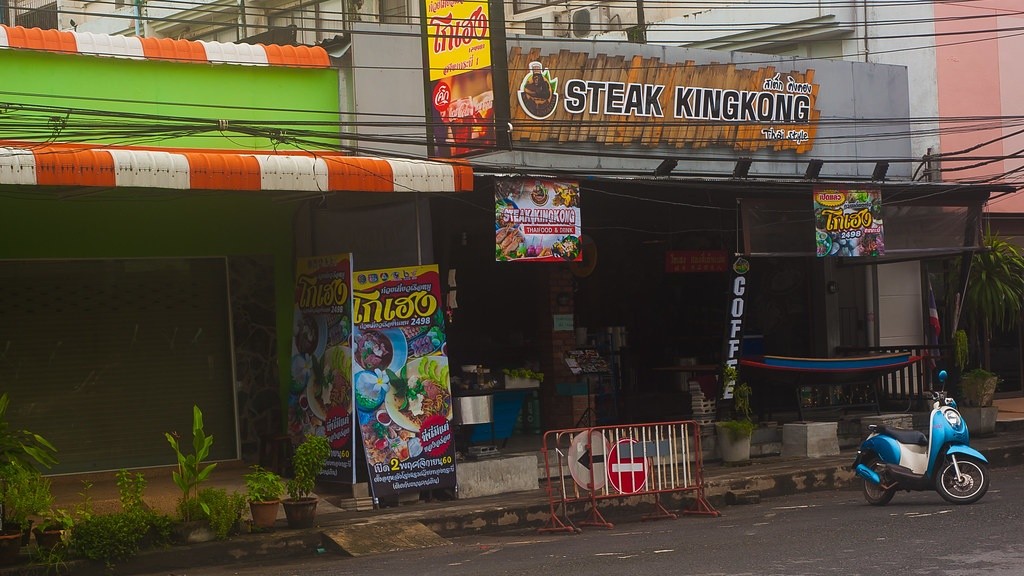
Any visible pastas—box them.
[409,394,443,426]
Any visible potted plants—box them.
[0,391,60,566]
[32,480,93,557]
[281,432,332,528]
[164,404,219,544]
[242,465,287,530]
[0,461,53,546]
[201,486,246,539]
[961,368,1004,407]
[715,421,753,462]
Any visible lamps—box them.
[872,162,889,184]
[654,159,680,178]
[804,158,825,181]
[734,158,753,181]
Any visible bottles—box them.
[477,365,484,385]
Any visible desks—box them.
[452,386,496,447]
[652,363,718,423]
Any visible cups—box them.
[473,90,495,144]
[448,96,474,149]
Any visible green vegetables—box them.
[356,391,377,407]
[495,199,508,214]
[371,421,397,447]
[495,246,527,261]
[311,351,335,398]
[385,364,427,411]
[563,234,580,258]
[502,368,544,383]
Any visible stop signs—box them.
[606,437,651,495]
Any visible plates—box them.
[407,332,446,360]
[376,410,391,426]
[354,370,385,412]
[385,356,452,432]
[354,326,408,374]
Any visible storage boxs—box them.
[713,334,765,358]
[503,367,545,388]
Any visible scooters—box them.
[852,371,991,506]
